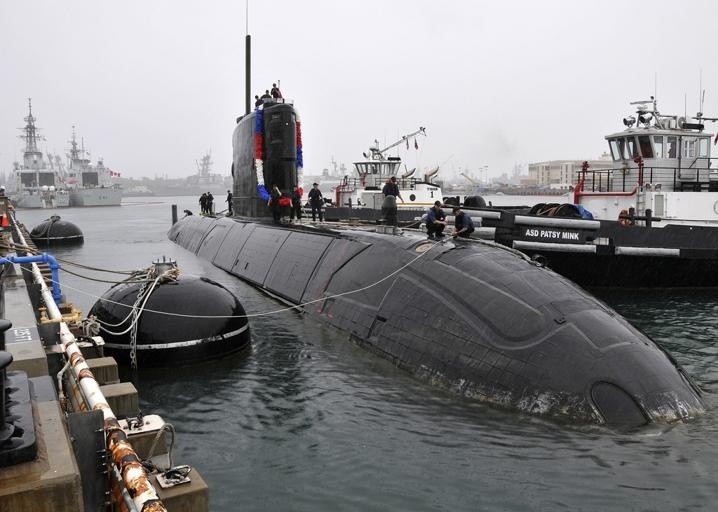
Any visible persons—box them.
[260,89,272,104]
[289,185,302,222]
[425,201,448,238]
[270,83,281,99]
[451,205,475,239]
[307,183,324,223]
[266,184,283,221]
[225,189,232,212]
[198,191,214,214]
[382,175,405,205]
[254,95,264,108]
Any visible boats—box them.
[325,126,444,228]
[441,70,718,288]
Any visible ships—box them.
[5,96,124,209]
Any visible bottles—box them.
[313,182,318,185]
[433,200,442,206]
[391,176,397,181]
[452,207,460,212]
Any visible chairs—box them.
[434,232,445,237]
[428,231,434,238]
